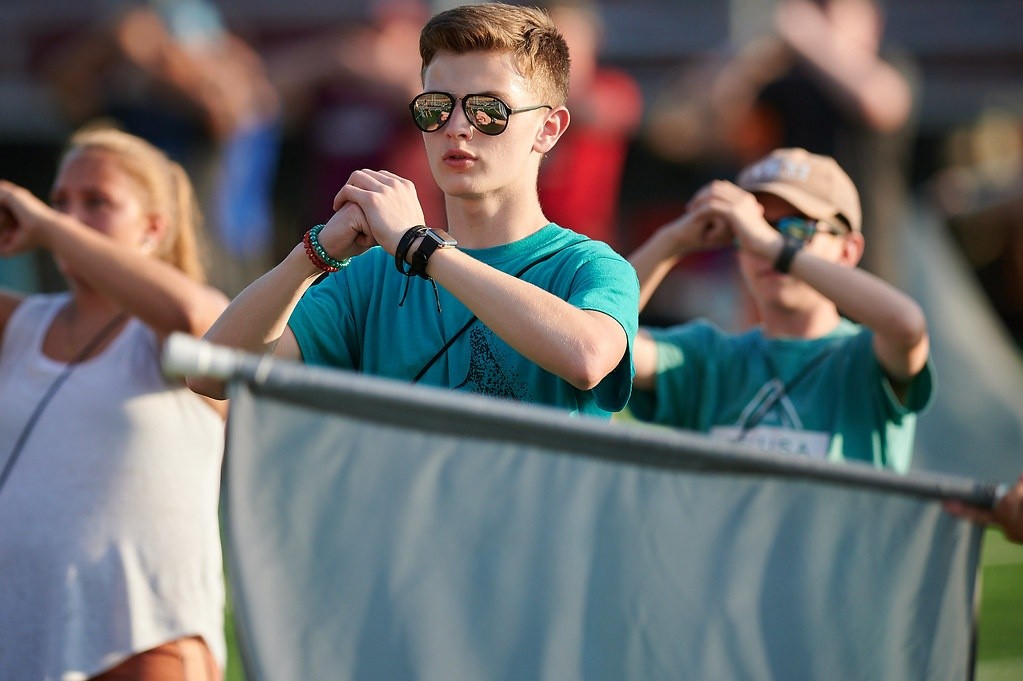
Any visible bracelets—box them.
[395,225,428,276]
[309,223,352,268]
[302,230,340,273]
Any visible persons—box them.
[1,124,230,681]
[623,145,937,476]
[184,1,638,424]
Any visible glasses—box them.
[409,92,552,136]
[767,214,842,241]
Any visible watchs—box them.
[775,236,802,273]
[412,228,458,280]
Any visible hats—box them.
[736,147,862,234]
